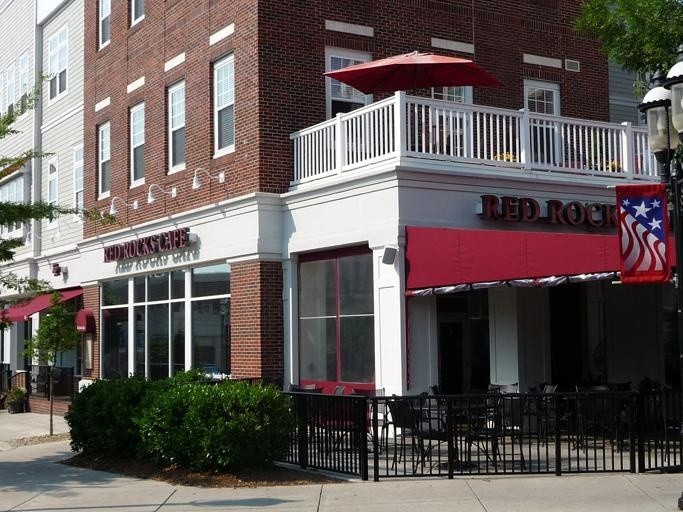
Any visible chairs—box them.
[288,378,683,471]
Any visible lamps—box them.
[110,167,225,215]
[381,244,398,265]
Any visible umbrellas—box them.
[320,50,506,101]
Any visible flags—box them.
[614,182,671,285]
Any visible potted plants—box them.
[6,386,28,413]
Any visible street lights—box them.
[638,43,682,312]
